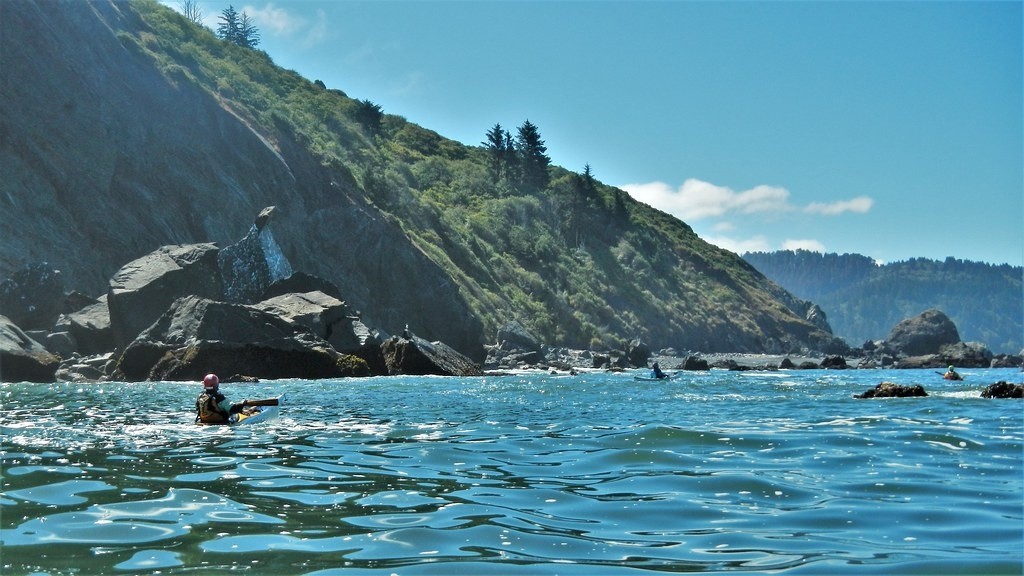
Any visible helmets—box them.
[948,365,954,371]
[204,374,219,389]
[652,363,659,368]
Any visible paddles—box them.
[233,398,279,406]
[935,371,945,376]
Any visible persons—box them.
[944,365,960,380]
[651,363,662,378]
[196,374,248,422]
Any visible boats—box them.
[634,370,684,382]
[235,393,288,426]
